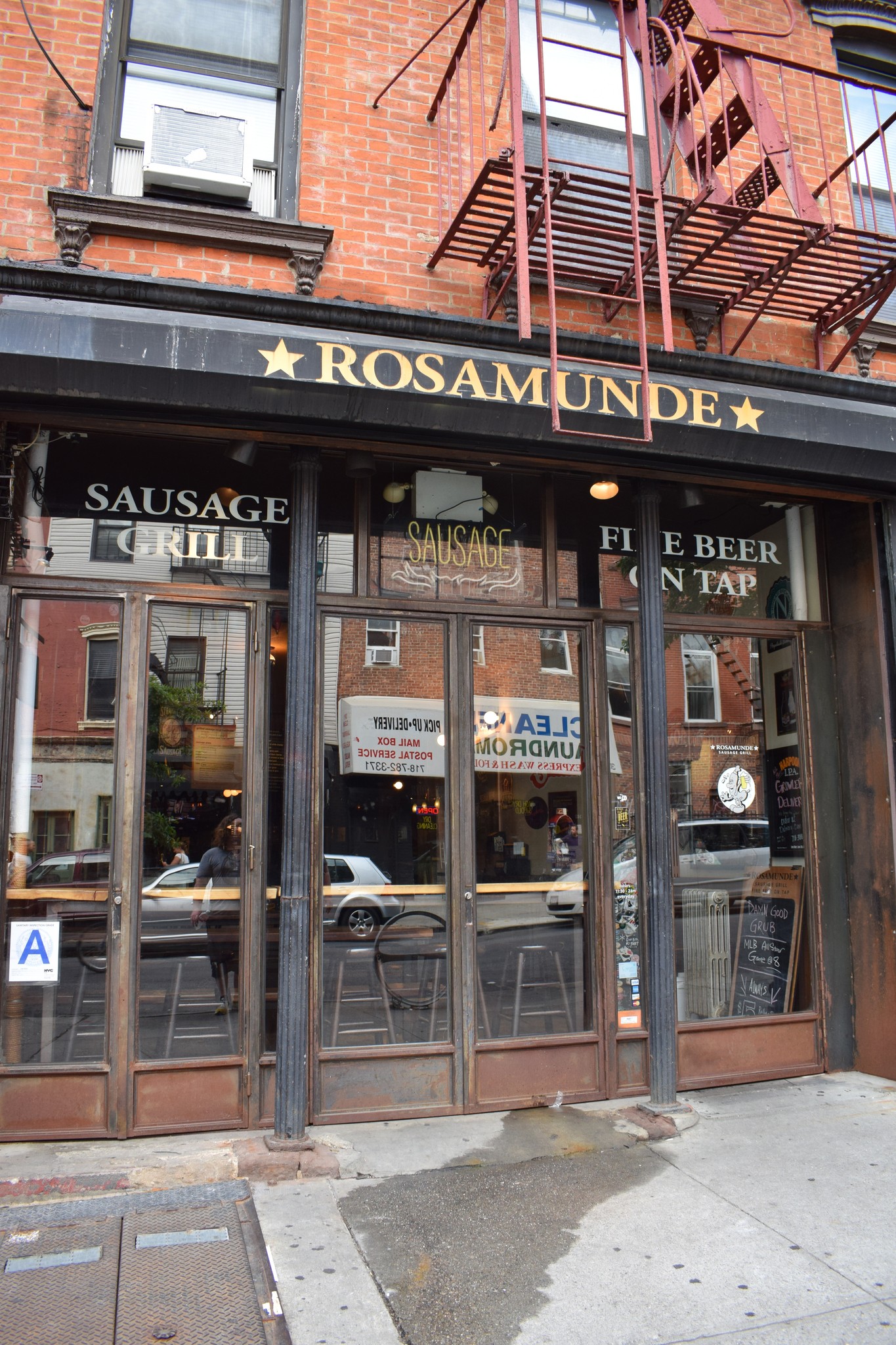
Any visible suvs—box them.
[546,817,770,926]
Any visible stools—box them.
[65,954,118,1064]
[323,944,399,1049]
[414,940,494,1047]
[493,938,579,1039]
[154,952,238,1058]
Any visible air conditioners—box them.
[140,99,255,201]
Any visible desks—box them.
[47,924,437,1062]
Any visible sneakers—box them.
[215,1001,229,1015]
[232,995,239,1010]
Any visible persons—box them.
[190,813,242,1016]
[555,823,583,871]
[323,855,334,913]
[9,839,36,881]
[161,841,190,867]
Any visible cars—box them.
[322,852,405,943]
[6,845,110,954]
[140,862,214,945]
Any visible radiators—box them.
[682,888,734,1018]
